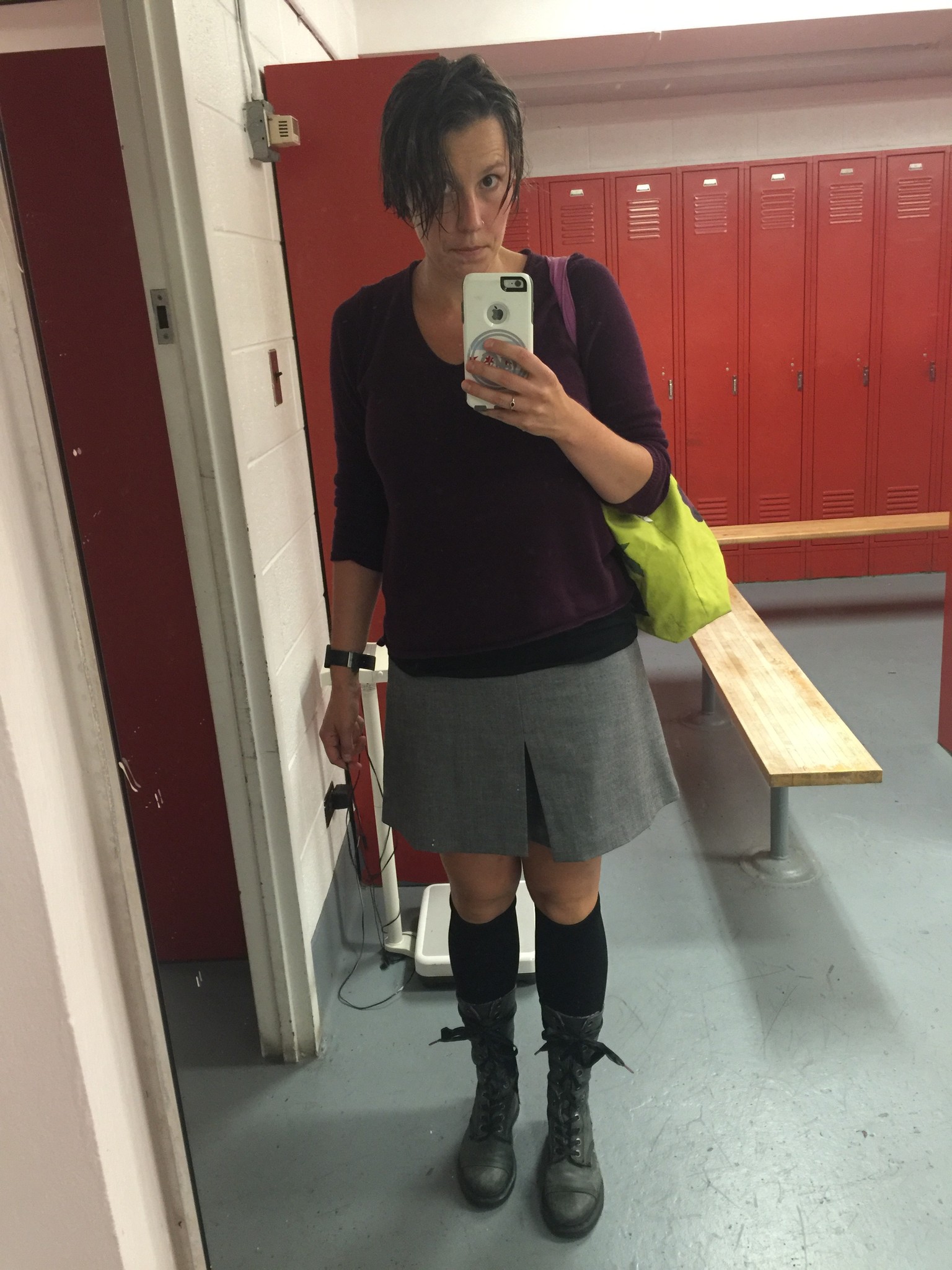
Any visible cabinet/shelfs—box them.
[267,54,440,888]
[502,143,952,582]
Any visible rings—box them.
[510,394,515,410]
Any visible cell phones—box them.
[463,272,533,415]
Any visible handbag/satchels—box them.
[546,253,729,642]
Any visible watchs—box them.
[324,644,376,671]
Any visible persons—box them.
[319,54,680,1240]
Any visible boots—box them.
[428,986,521,1210]
[535,1003,633,1240]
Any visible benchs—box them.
[689,581,883,889]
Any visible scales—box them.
[320,642,535,977]
